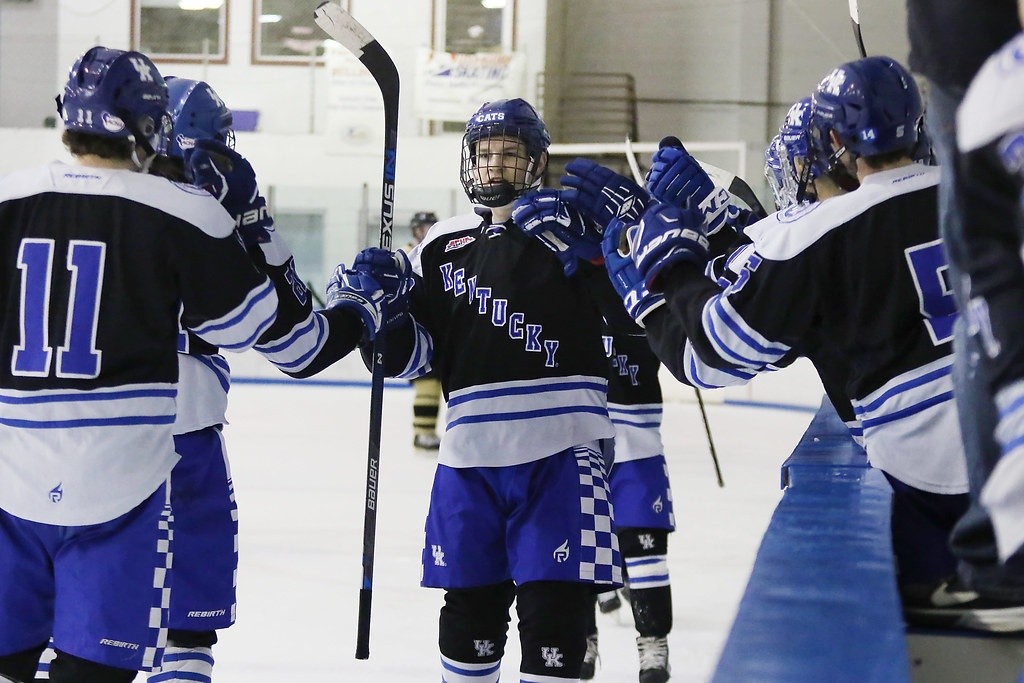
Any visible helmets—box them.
[763,136,801,211]
[810,57,930,185]
[779,97,825,204]
[56,45,173,170]
[459,99,552,206]
[160,76,235,179]
[410,211,438,238]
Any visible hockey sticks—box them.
[311,1,401,660]
[623,135,724,489]
[849,1,868,58]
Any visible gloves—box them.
[644,135,761,238]
[352,246,412,332]
[180,135,275,246]
[320,265,387,342]
[559,157,654,233]
[601,217,666,331]
[512,186,604,277]
[632,201,712,294]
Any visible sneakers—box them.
[578,628,601,682]
[635,634,671,683]
[596,585,622,623]
[620,579,632,605]
[897,573,1024,634]
[414,430,441,454]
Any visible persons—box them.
[360,1,1024,683]
[31,75,385,682]
[402,211,442,450]
[0,45,279,683]
